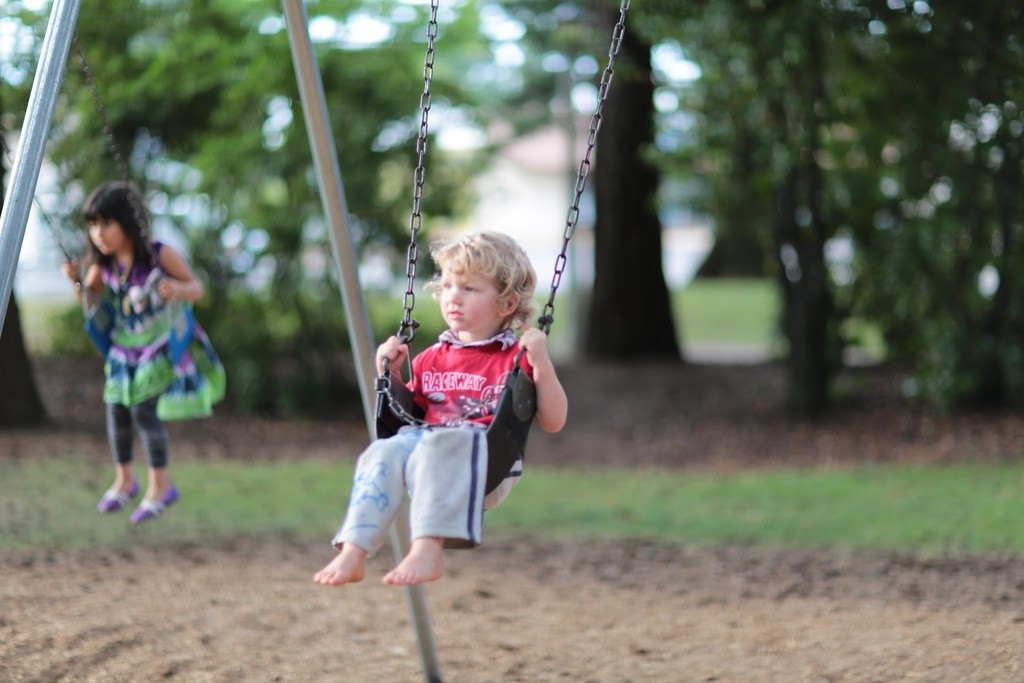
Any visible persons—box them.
[312,230,567,586]
[65,180,204,525]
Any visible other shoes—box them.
[130,485,177,522]
[99,481,138,511]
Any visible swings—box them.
[368,0,630,500]
[4,38,229,424]
